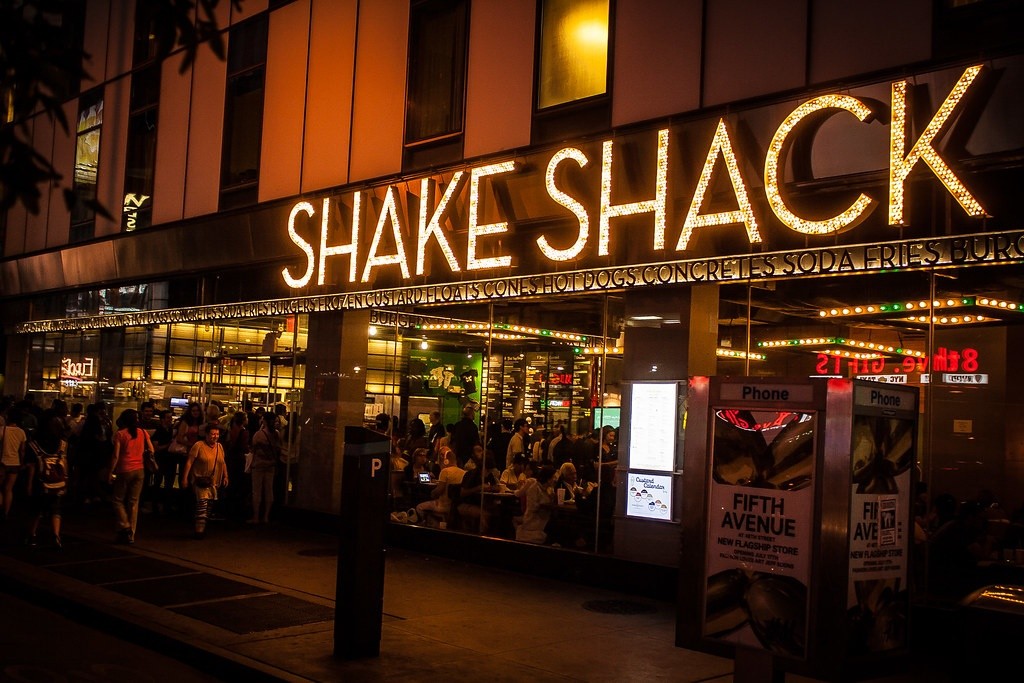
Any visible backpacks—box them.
[25,437,69,501]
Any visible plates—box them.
[564,500,575,504]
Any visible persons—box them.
[913,492,969,579]
[0,392,619,550]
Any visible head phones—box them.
[444,450,450,465]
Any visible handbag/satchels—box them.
[168,423,189,454]
[142,449,158,478]
[196,475,212,489]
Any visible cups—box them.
[499,484,506,493]
[572,486,583,498]
[557,489,565,504]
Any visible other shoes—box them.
[247,517,269,527]
[115,523,135,546]
[52,535,62,550]
[27,534,39,548]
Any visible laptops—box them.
[418,471,436,485]
